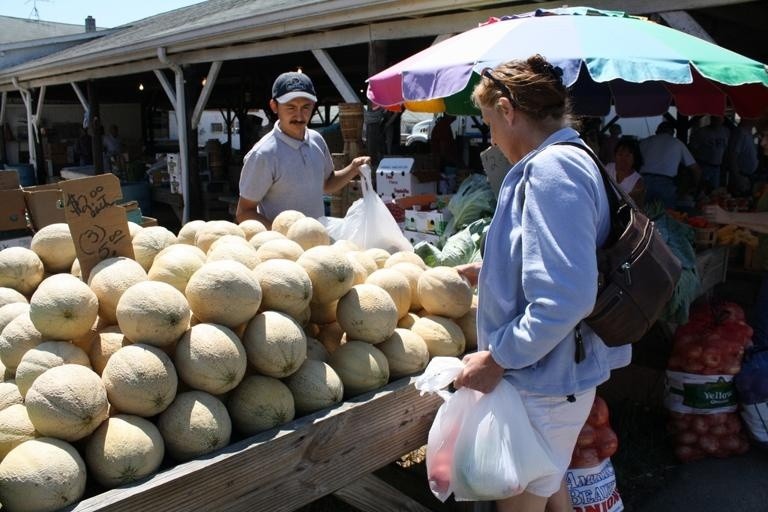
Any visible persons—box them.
[447,61,611,512]
[234,70,373,230]
[419,104,768,244]
[703,114,768,354]
[101,125,123,154]
[73,127,93,166]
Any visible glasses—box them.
[481,67,519,110]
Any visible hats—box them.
[271,71,318,104]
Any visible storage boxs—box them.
[346,157,461,257]
[0,164,158,256]
[167,151,212,194]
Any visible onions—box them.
[664,296,755,466]
[570,396,618,468]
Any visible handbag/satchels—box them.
[520,141,682,364]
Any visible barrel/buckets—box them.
[338,102,366,141]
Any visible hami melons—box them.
[0,210,478,512]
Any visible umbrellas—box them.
[366,7,768,126]
